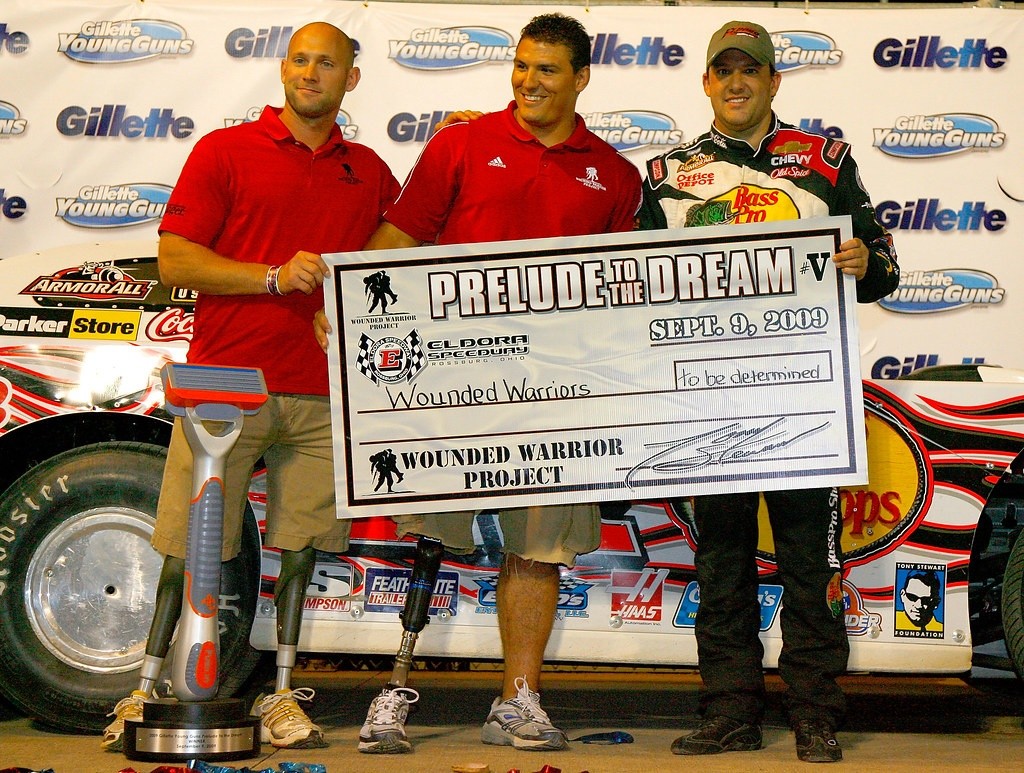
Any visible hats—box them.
[707,21,776,71]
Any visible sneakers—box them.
[481,677,568,749]
[792,708,843,762]
[259,686,330,748]
[99,690,154,752]
[357,685,420,754]
[672,716,764,754]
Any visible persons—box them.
[633,22,901,761]
[101,22,484,754]
[312,14,642,753]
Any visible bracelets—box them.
[266,264,284,296]
